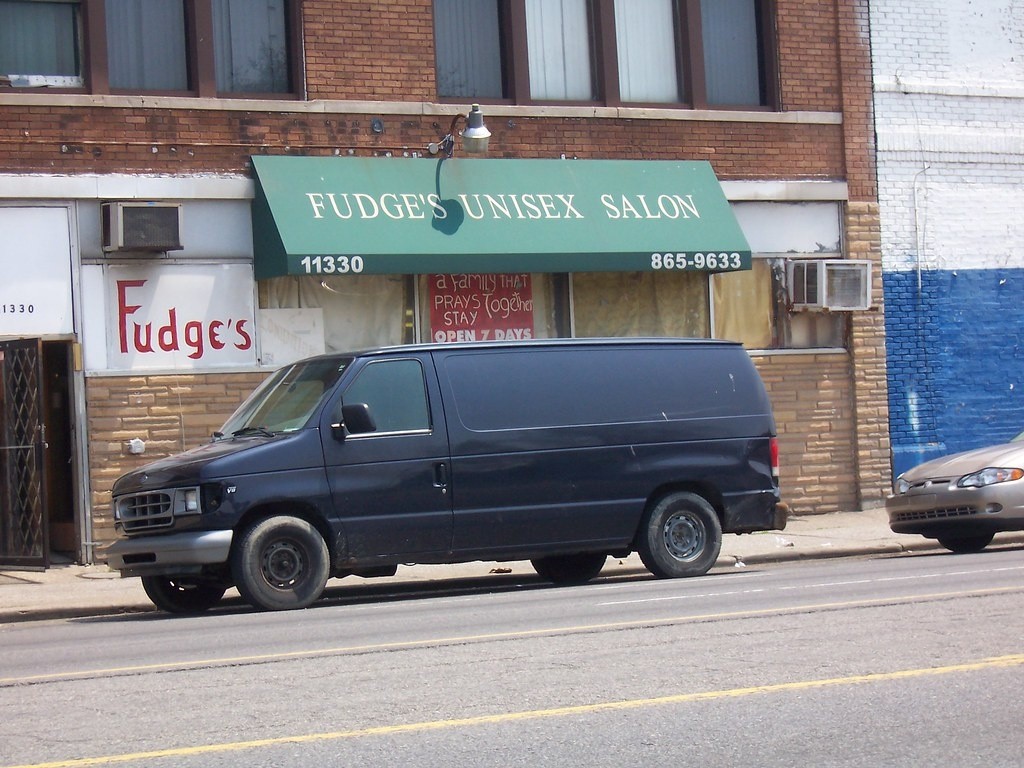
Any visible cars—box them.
[885,431,1024,552]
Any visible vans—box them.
[106,337,788,612]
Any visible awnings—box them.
[250,155,752,281]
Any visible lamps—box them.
[444,103,492,153]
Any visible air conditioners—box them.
[101,201,184,253]
[786,259,872,313]
